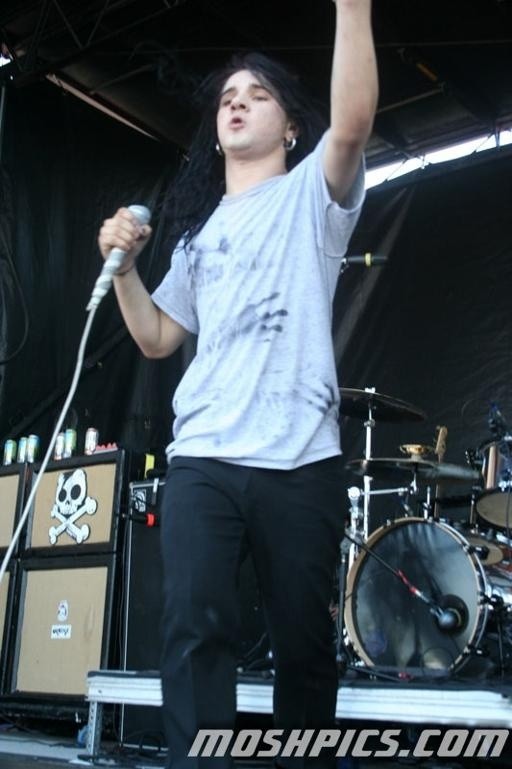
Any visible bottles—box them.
[4,439,16,466]
[54,432,65,461]
[85,428,99,456]
[26,435,39,462]
[18,437,28,464]
[64,429,76,458]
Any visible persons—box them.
[97,0,379,769]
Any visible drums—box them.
[468,433,512,531]
[343,517,512,684]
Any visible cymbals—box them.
[348,455,432,474]
[339,385,428,421]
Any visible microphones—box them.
[86,201,153,312]
[346,249,391,270]
[130,510,161,530]
[414,590,459,630]
